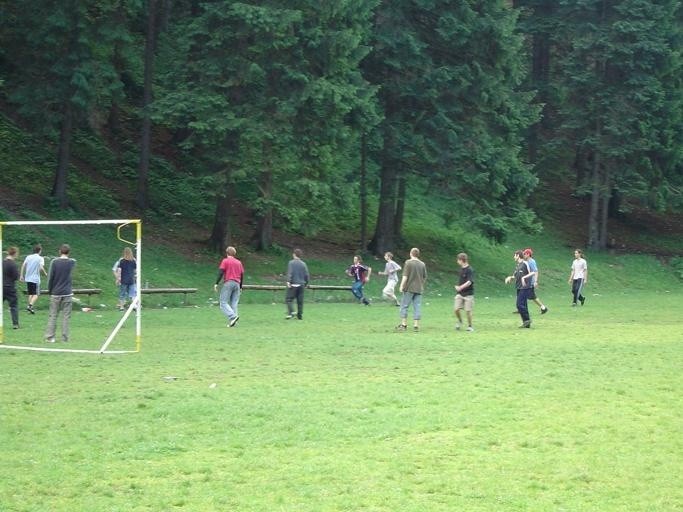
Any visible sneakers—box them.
[230,316,239,327]
[26,306,34,314]
[395,324,407,329]
[541,308,547,314]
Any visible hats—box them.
[524,249,531,257]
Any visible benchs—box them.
[236,282,287,301]
[139,287,197,306]
[305,283,365,300]
[22,287,103,307]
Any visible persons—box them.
[282,247,308,320]
[2,246,19,329]
[18,244,48,313]
[453,253,475,332]
[215,247,244,328]
[345,254,373,306]
[377,251,401,305]
[392,247,426,332]
[114,246,140,312]
[510,248,548,315]
[568,248,589,307]
[43,244,76,343]
[110,255,136,309]
[503,250,535,329]
